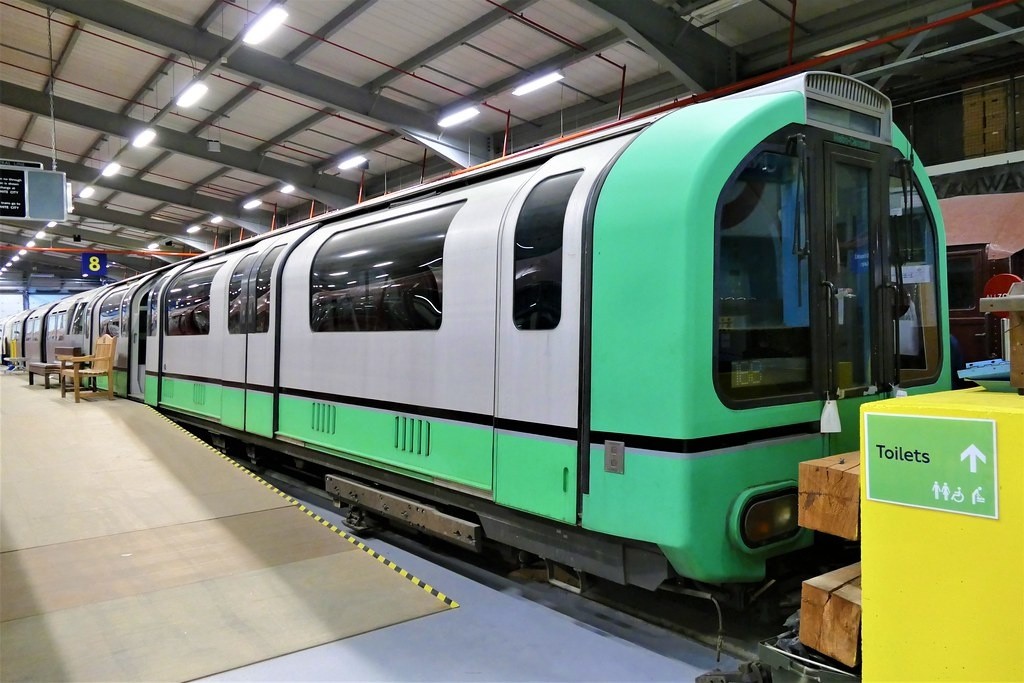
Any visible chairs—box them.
[57,333,117,403]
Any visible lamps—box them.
[242,3,289,46]
[186,223,204,234]
[1,186,96,272]
[101,161,121,178]
[511,68,567,96]
[437,105,481,128]
[175,80,209,108]
[338,154,368,170]
[132,128,157,148]
[243,199,263,210]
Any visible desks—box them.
[4,358,30,373]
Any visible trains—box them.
[1,70,954,610]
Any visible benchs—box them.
[29,347,83,389]
